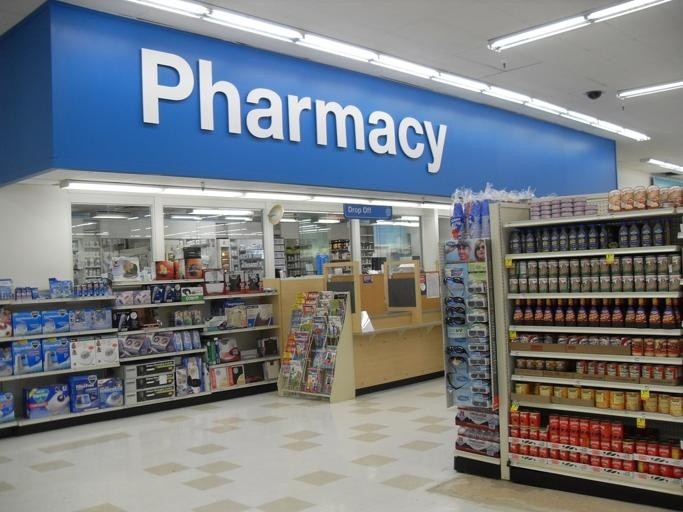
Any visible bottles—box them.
[507,217,665,254]
[512,298,682,330]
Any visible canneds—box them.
[508,253,681,293]
[509,333,683,478]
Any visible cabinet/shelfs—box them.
[2,231,445,439]
[442,187,682,511]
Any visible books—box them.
[279,291,347,395]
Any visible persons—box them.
[443,240,455,253]
[473,239,484,262]
[455,240,473,262]
[113,259,137,276]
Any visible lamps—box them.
[59,178,453,211]
[643,159,683,173]
[126,1,650,141]
[485,1,671,53]
[618,81,681,100]
